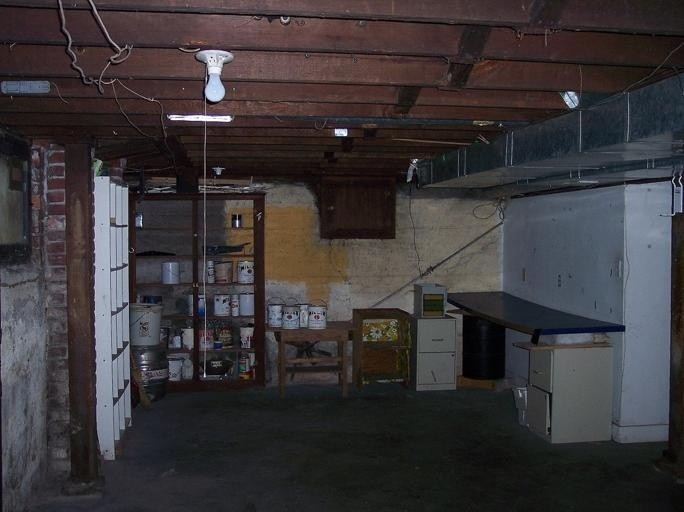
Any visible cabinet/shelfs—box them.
[130,191,267,391]
[95,175,130,462]
[416,312,456,393]
[354,309,412,390]
[320,184,397,239]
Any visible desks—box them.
[448,292,624,447]
[269,321,356,401]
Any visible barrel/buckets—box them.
[130,303,163,346]
[295,302,312,328]
[309,298,328,330]
[267,296,285,328]
[166,357,184,381]
[213,293,231,316]
[215,261,233,283]
[237,260,255,284]
[162,262,180,284]
[184,294,206,316]
[131,346,168,402]
[281,296,300,329]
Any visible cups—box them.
[214,341,222,350]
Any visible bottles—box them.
[245,356,251,373]
[232,214,242,228]
[207,260,215,284]
[230,295,240,318]
[198,295,205,317]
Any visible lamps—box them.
[194,49,234,102]
